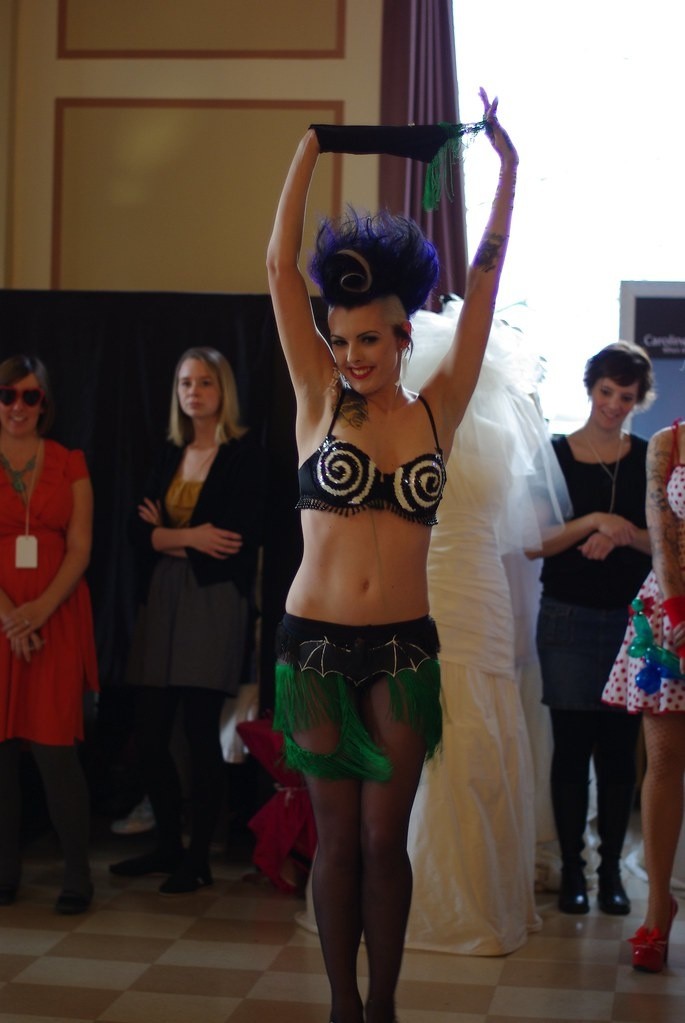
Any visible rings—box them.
[24,621,30,626]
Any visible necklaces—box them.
[0,451,36,491]
[582,431,623,513]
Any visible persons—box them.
[596,412,684,972]
[109,346,282,892]
[523,336,651,916]
[259,89,519,1022]
[290,292,572,957]
[0,352,98,915]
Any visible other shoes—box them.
[110,853,181,876]
[0,890,16,904]
[56,885,94,913]
[157,875,215,895]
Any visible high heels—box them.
[629,895,678,974]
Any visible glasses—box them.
[0,386,45,406]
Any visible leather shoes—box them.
[598,874,629,914]
[560,876,588,915]
